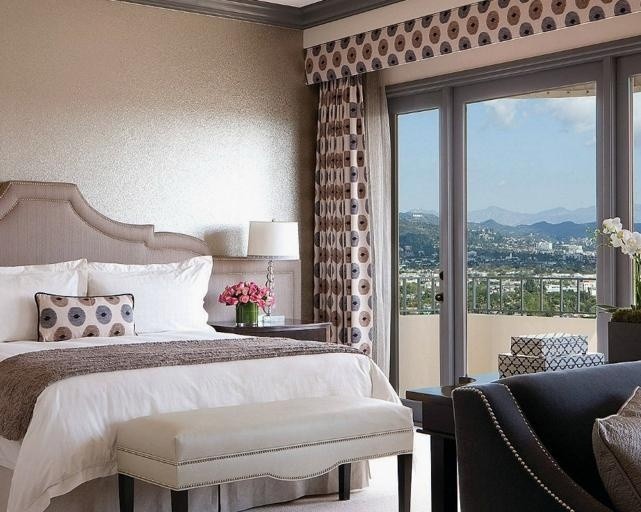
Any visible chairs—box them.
[451,358,640,512]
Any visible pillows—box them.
[591,383,641,512]
[0,258,88,343]
[89,255,215,334]
[34,290,136,342]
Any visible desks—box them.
[406,375,499,512]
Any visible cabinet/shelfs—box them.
[595,309,640,364]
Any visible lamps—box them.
[247,219,300,321]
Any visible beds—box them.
[1,178,401,510]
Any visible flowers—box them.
[219,281,275,309]
[591,215,641,302]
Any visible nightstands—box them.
[214,319,332,343]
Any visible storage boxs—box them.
[511,333,587,357]
[498,352,605,382]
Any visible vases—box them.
[237,301,258,326]
[633,257,641,310]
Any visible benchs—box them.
[118,397,415,511]
